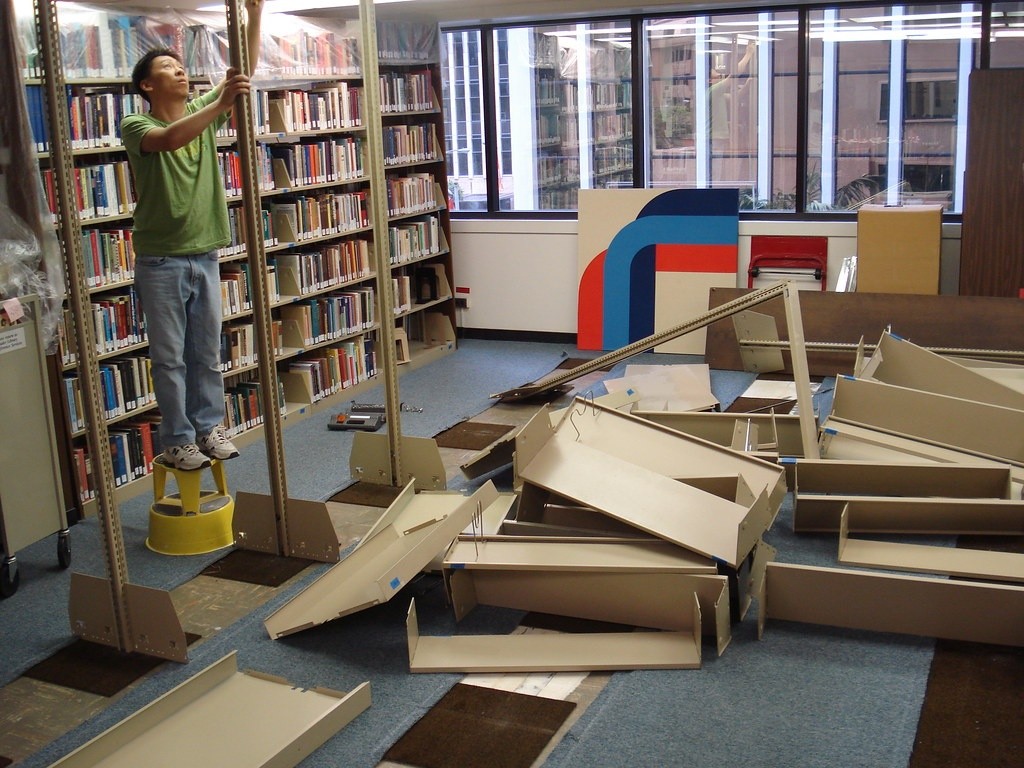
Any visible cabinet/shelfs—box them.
[0,0,458,523]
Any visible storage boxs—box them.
[856,204,944,295]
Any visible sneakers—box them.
[163,444,211,471]
[196,427,240,460]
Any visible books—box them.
[10,12,446,506]
[532,59,687,213]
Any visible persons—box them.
[706,40,761,187]
[118,0,263,472]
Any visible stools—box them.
[151,452,229,516]
